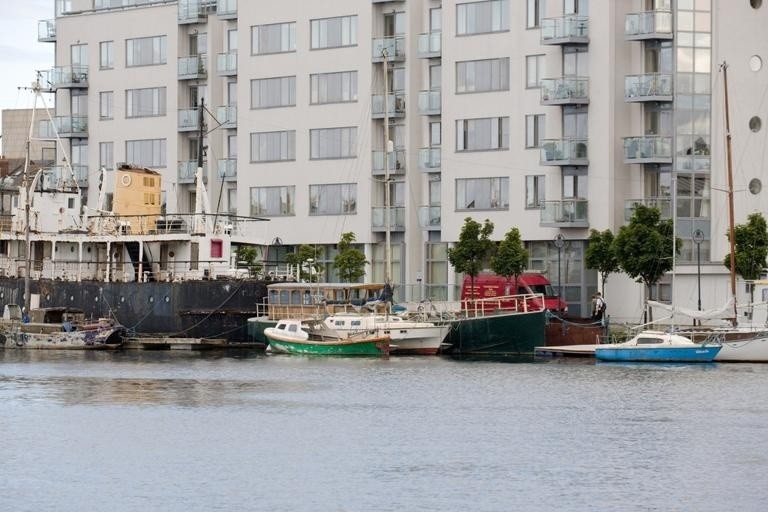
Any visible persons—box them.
[594,292,604,322]
[22,313,29,323]
[590,296,597,321]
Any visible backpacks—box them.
[599,298,607,313]
